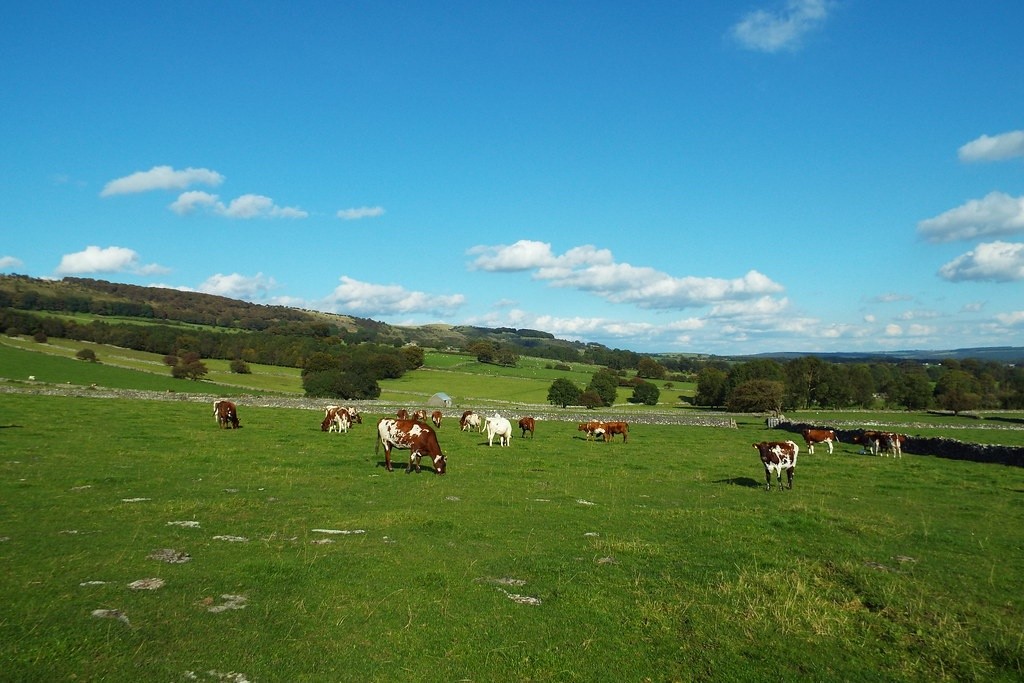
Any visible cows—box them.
[752,440,800,491]
[853,432,905,457]
[800,427,839,455]
[212,401,240,429]
[375,408,535,476]
[578,421,630,442]
[320,406,362,434]
